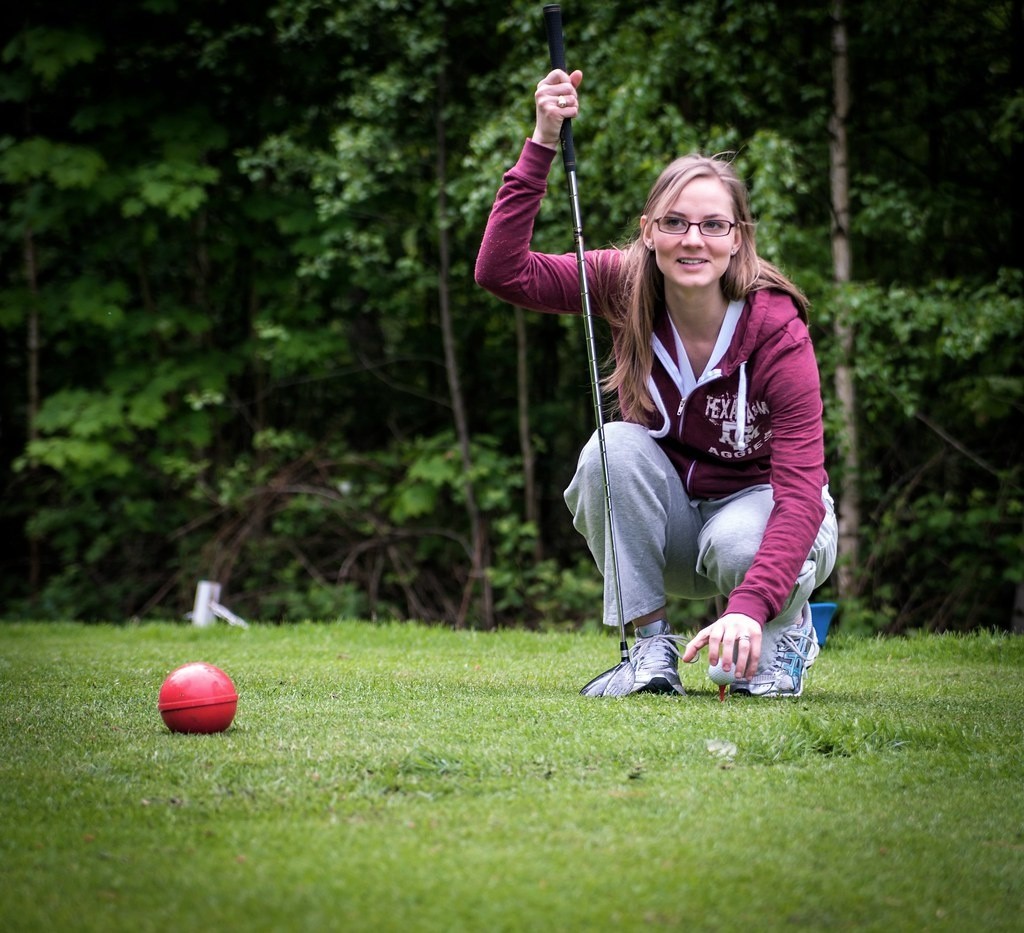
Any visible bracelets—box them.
[532,136,559,143]
[558,94,568,108]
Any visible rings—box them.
[738,635,749,641]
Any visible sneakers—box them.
[629,628,700,694]
[729,598,819,698]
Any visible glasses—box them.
[652,216,739,237]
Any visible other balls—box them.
[159,660,238,732]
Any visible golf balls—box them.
[707,657,738,688]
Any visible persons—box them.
[474,69,838,696]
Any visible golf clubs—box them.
[540,1,633,707]
